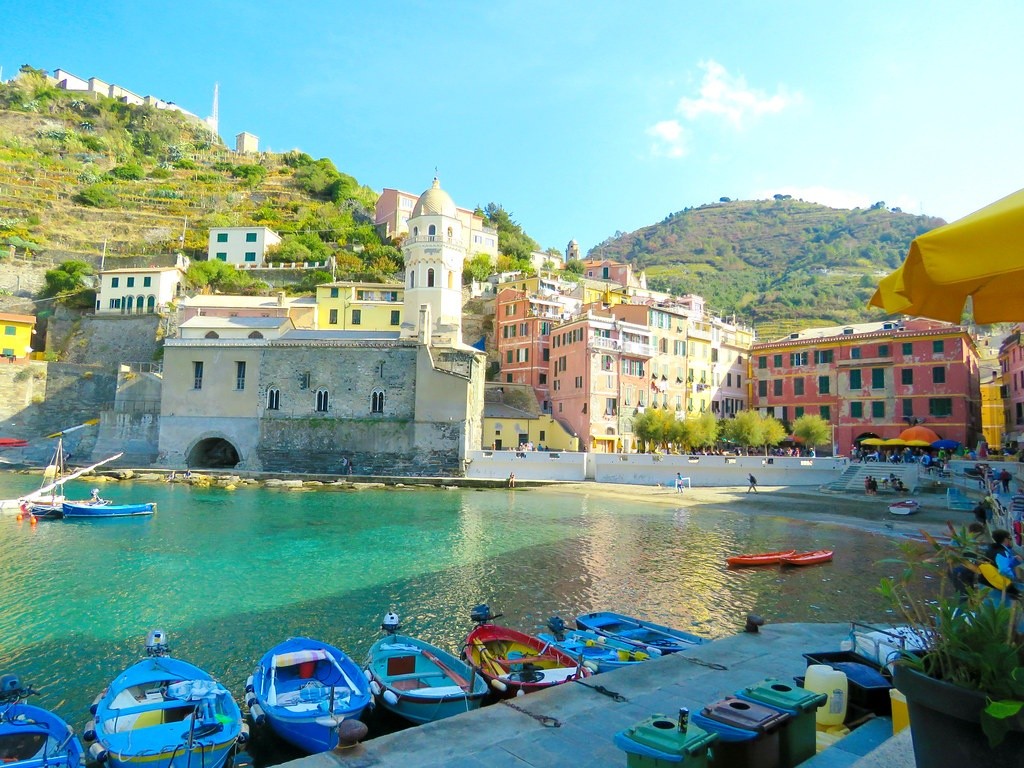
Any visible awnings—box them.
[897,425,940,443]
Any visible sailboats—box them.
[0,435,125,525]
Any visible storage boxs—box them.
[802,650,894,714]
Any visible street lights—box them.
[832,424,838,456]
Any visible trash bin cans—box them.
[690,695,790,768]
[614,713,721,768]
[733,676,828,768]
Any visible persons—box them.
[508,472,516,488]
[984,464,994,492]
[681,446,816,463]
[994,498,1007,517]
[881,477,888,488]
[986,530,1023,604]
[183,468,193,480]
[519,441,534,458]
[545,446,549,451]
[166,471,176,482]
[890,472,904,492]
[991,480,1000,497]
[617,438,623,453]
[583,443,588,452]
[998,468,1013,493]
[865,475,878,496]
[537,444,543,451]
[338,455,353,475]
[746,473,758,494]
[677,472,684,493]
[977,463,986,490]
[949,522,984,606]
[851,445,1010,474]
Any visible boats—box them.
[0,672,89,768]
[243,636,375,753]
[725,549,797,569]
[61,501,158,519]
[888,500,921,515]
[362,611,491,726]
[458,603,595,698]
[83,628,251,768]
[779,548,835,568]
[574,610,704,656]
[535,616,662,675]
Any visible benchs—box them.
[560,639,596,648]
[104,697,210,720]
[1,755,68,768]
[386,671,447,682]
[494,657,549,665]
[616,628,651,637]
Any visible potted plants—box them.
[868,523,1024,768]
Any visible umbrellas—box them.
[860,437,963,448]
